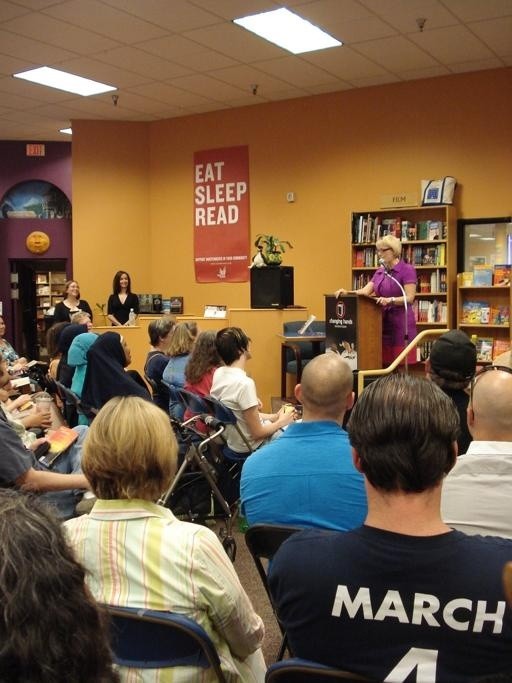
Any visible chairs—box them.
[254,655,379,681]
[1,356,261,568]
[92,596,228,683]
[279,319,326,400]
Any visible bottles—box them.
[136,293,184,314]
[128,308,138,325]
[235,496,250,532]
[470,333,479,346]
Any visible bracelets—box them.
[389,296,395,305]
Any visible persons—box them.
[1,312,511,681]
[55,279,94,325]
[3,188,17,217]
[106,270,140,326]
[336,234,418,366]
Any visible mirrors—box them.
[458,214,512,278]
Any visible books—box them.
[353,214,511,363]
[139,293,184,313]
[35,273,65,315]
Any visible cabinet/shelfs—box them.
[352,204,456,377]
[36,271,66,337]
[457,286,511,374]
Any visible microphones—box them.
[379,258,390,274]
[96,302,108,325]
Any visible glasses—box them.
[374,248,390,256]
[470,364,512,419]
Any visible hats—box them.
[430,331,478,384]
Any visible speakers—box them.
[250,264,293,307]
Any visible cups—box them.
[282,402,297,424]
[36,397,55,415]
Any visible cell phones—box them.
[34,441,51,460]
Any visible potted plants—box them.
[254,233,294,264]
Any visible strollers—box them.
[157,410,238,564]
